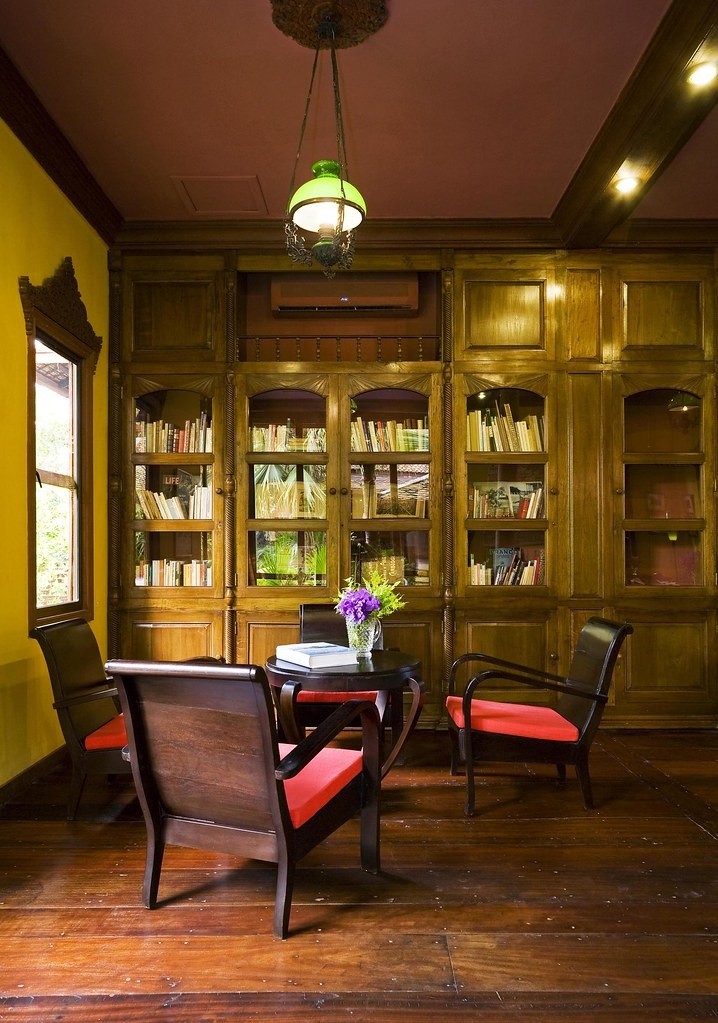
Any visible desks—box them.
[262,646,429,780]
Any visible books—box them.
[275,642,359,669]
[134,399,547,587]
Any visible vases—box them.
[344,615,383,658]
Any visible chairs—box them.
[100,657,392,941]
[28,616,231,826]
[441,615,638,819]
[298,600,406,744]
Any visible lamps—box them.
[667,389,702,414]
[268,0,391,280]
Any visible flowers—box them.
[329,565,411,623]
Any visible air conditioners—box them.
[268,272,421,314]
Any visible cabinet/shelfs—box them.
[118,360,718,731]
